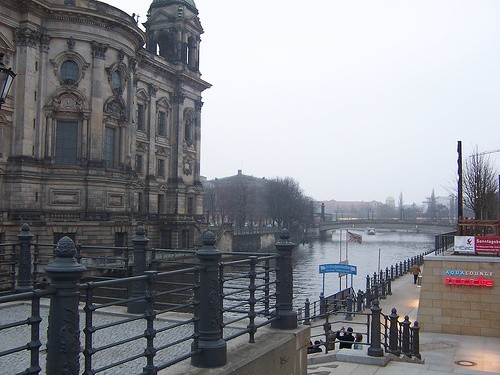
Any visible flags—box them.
[347,231,362,245]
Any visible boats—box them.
[366,228,376,235]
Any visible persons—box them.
[320,322,336,350]
[336,326,355,349]
[307,340,316,354]
[410,262,421,284]
[354,333,363,350]
[314,340,322,352]
